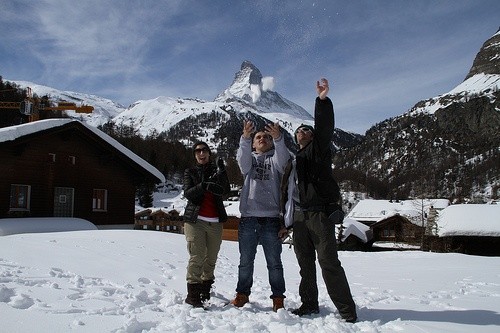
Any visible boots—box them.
[201,280,213,304]
[185,282,204,308]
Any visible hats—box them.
[294,125,314,146]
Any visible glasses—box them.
[295,127,313,135]
[194,146,208,154]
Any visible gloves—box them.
[207,182,224,196]
[215,156,225,172]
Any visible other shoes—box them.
[344,314,357,323]
[292,304,320,317]
[272,298,285,312]
[230,294,248,308]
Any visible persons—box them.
[232,120,291,313]
[279,78,358,323]
[183,141,231,310]
